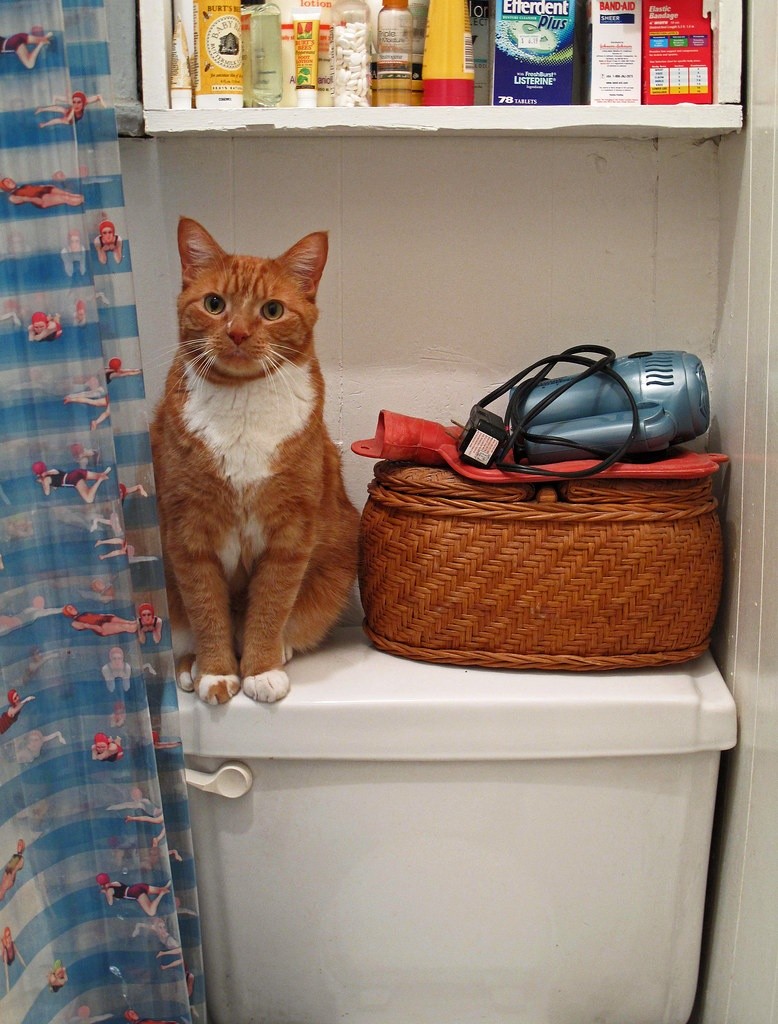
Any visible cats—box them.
[148,211,363,710]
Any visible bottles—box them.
[376,0,413,107]
[193,0,243,110]
[331,1,371,106]
[239,0,284,108]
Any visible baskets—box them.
[358,460,724,672]
